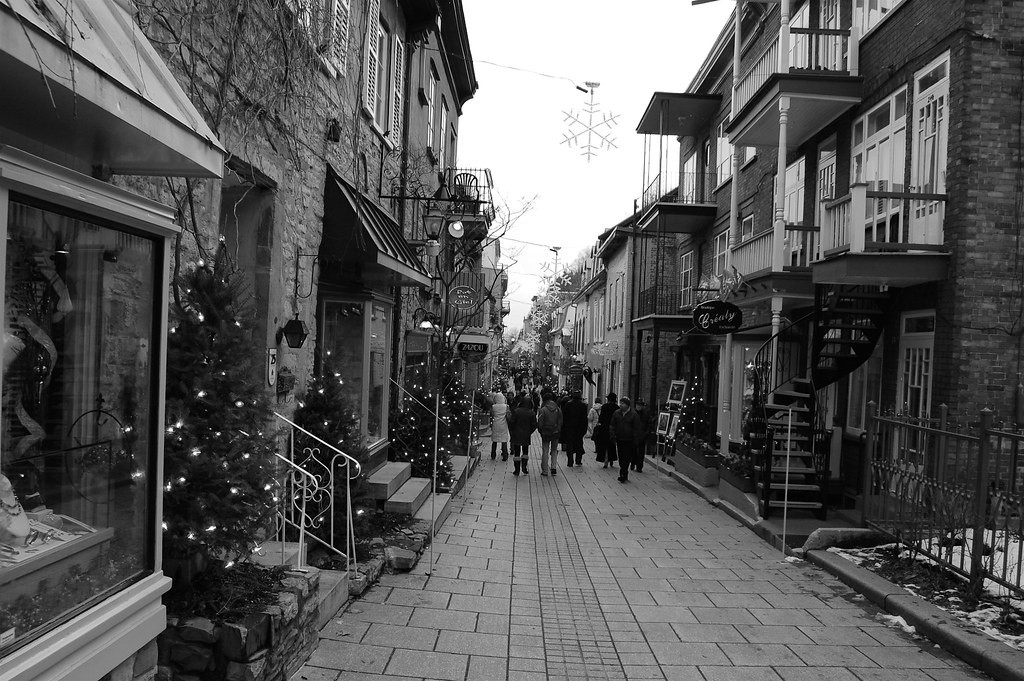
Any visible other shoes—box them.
[567,463,573,467]
[617,477,628,483]
[541,473,548,476]
[603,463,608,468]
[636,468,642,473]
[610,462,613,467]
[630,463,635,470]
[510,452,514,455]
[576,461,582,465]
[551,469,557,476]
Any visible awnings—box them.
[325,162,433,291]
[0,0,228,181]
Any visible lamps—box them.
[276,312,309,348]
[594,367,601,373]
[448,201,466,238]
[412,307,433,329]
[644,336,675,343]
[405,212,447,256]
[433,165,453,199]
[676,326,697,342]
[326,116,343,142]
[426,311,439,328]
[65,411,142,504]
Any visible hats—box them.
[635,401,644,405]
[595,398,601,403]
[606,392,618,402]
[542,392,554,401]
[620,395,631,406]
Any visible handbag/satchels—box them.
[591,422,603,442]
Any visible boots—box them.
[501,452,508,461]
[521,455,529,474]
[513,457,521,475]
[491,452,496,460]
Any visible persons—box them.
[490,364,652,484]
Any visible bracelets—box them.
[40,528,55,543]
[25,530,39,546]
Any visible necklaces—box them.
[0,486,84,564]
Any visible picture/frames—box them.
[667,380,688,404]
[669,414,680,436]
[656,412,670,435]
